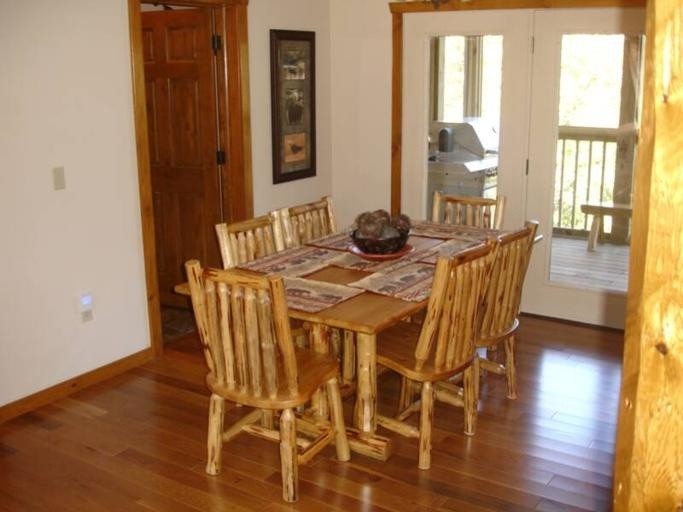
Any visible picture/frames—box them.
[267,28,316,185]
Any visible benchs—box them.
[582,200,630,252]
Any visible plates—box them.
[347,237,416,259]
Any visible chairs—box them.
[430,189,507,232]
[434,219,542,401]
[184,260,350,503]
[215,210,311,416]
[375,235,500,472]
[280,194,341,355]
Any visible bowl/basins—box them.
[350,226,410,255]
